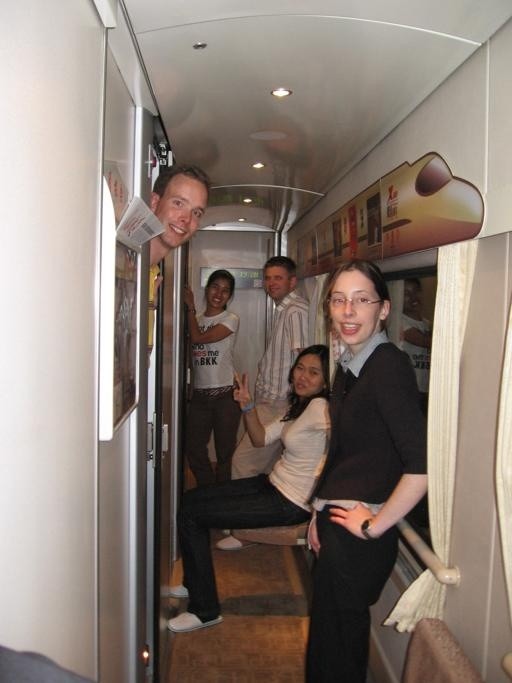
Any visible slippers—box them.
[167,609,225,633]
[215,534,260,551]
[170,583,191,599]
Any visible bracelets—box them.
[241,401,255,413]
[187,308,197,314]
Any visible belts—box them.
[193,385,236,397]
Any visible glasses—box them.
[327,294,383,306]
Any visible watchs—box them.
[361,516,376,540]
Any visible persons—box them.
[167,341,333,636]
[213,255,313,553]
[180,265,241,534]
[145,160,213,378]
[302,256,429,683]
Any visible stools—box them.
[232,509,327,556]
